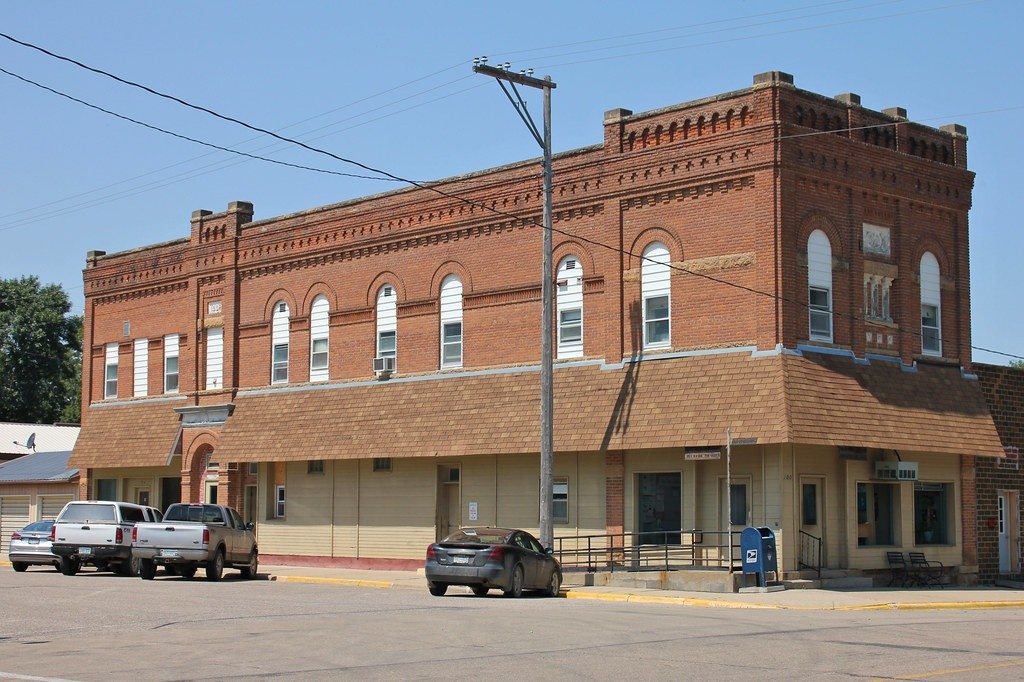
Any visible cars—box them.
[9,519,83,573]
[425,527,563,598]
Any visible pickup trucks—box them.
[133,503,259,580]
[52,501,164,576]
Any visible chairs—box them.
[886,552,921,588]
[909,553,945,589]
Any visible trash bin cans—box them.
[740,526,779,588]
